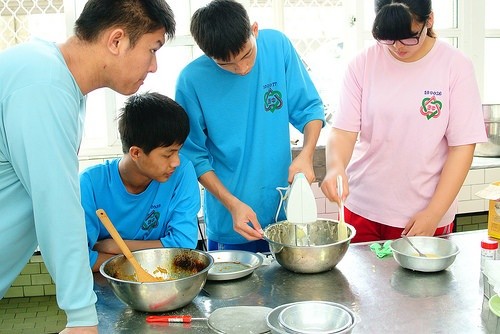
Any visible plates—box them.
[206,249,263,280]
[207,306,272,334]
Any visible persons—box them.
[80,92,202,271]
[174,0,325,254]
[316,0,488,244]
[0,1,175,334]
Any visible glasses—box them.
[377,14,427,45]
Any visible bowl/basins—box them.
[391,235,459,271]
[264,220,356,272]
[267,300,360,334]
[101,248,213,312]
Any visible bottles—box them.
[480,241,498,266]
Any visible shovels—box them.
[94,208,164,282]
[337,175,349,242]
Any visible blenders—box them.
[285,173,318,247]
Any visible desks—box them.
[114,228,489,334]
